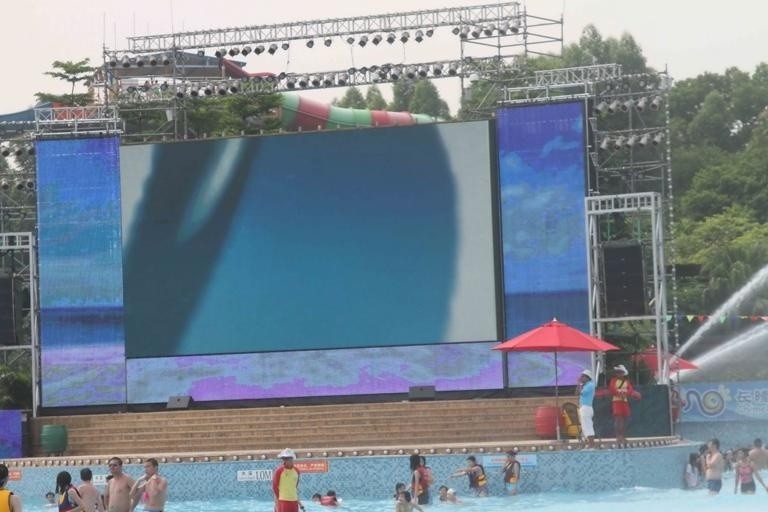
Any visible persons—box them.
[328,489,338,505]
[311,492,321,500]
[45,457,168,512]
[500,450,521,497]
[446,456,490,497]
[575,370,597,446]
[669,381,686,432]
[271,446,306,512]
[607,364,638,441]
[396,453,462,512]
[0,464,23,512]
[683,437,767,495]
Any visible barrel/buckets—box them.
[40,423,68,453]
[535,406,564,439]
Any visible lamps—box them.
[0,173,39,193]
[595,129,668,153]
[0,136,36,159]
[591,90,669,118]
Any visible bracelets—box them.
[299,506,305,509]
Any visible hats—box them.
[277,447,297,461]
[581,370,592,381]
[614,364,628,376]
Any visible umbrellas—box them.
[624,345,701,384]
[493,316,621,446]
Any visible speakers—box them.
[593,383,672,438]
[0,271,22,346]
[166,395,194,409]
[408,385,437,402]
[596,240,649,318]
